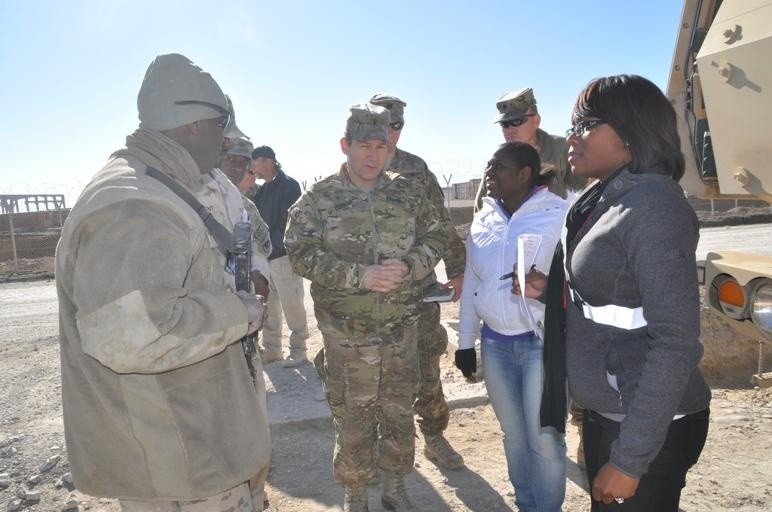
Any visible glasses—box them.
[566,119,607,139]
[389,121,403,130]
[174,100,231,130]
[500,114,536,128]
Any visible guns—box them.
[231,220,256,392]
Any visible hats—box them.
[229,138,254,157]
[494,87,535,122]
[136,53,227,132]
[346,104,391,140]
[369,93,406,125]
[252,145,276,160]
[223,94,249,138]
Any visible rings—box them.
[613,495,625,506]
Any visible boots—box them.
[381,469,421,512]
[260,343,284,366]
[422,427,465,470]
[343,485,369,511]
[283,346,309,368]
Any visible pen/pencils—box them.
[499,264,537,281]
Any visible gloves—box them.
[453,348,478,377]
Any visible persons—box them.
[250,145,309,368]
[313,91,466,486]
[194,93,271,511]
[474,86,592,472]
[280,102,447,512]
[454,141,589,512]
[54,52,272,512]
[512,74,712,512]
[222,98,273,265]
[241,163,265,202]
